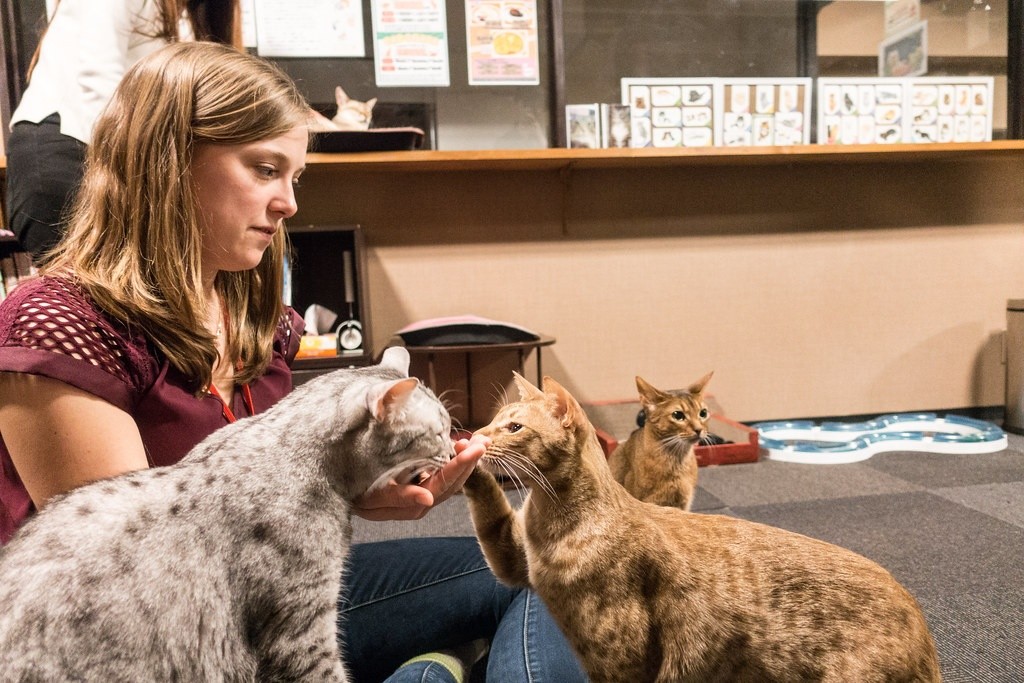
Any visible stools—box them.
[406,336,555,430]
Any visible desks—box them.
[270,140,1024,424]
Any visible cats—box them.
[463,369,945,683]
[0,346,458,683]
[608,371,714,513]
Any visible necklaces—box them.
[215,313,223,336]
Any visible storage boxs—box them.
[581,392,761,465]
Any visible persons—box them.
[0,1,590,682]
